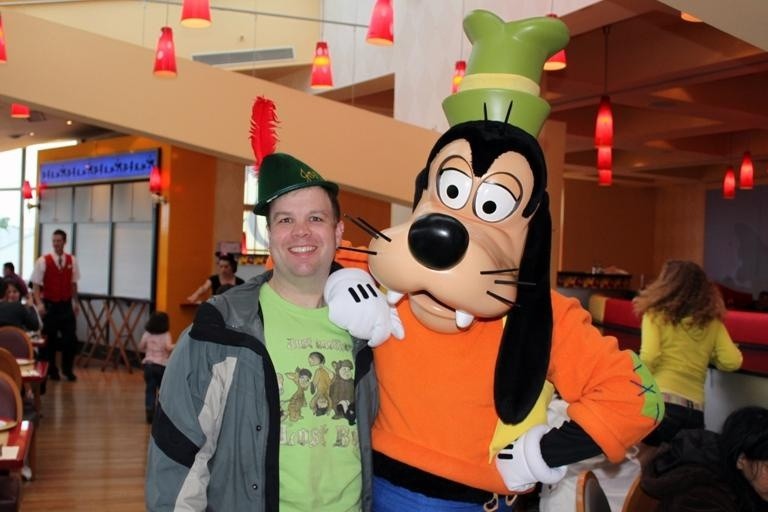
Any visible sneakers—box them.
[49,372,77,381]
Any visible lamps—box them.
[1,41,10,67]
[36,180,49,196]
[309,40,335,92]
[9,98,32,118]
[452,60,469,96]
[20,180,34,199]
[151,25,177,81]
[722,168,738,200]
[148,164,162,195]
[367,1,396,47]
[737,149,756,191]
[540,12,567,72]
[182,1,213,30]
[593,95,615,186]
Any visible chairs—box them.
[0,325,39,512]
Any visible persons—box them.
[185,251,245,304]
[639,404,768,512]
[628,259,744,447]
[138,311,176,425]
[143,151,381,512]
[0,228,82,420]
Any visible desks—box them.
[72,293,151,374]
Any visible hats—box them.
[254,153,338,215]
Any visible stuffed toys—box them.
[323,8,667,512]
